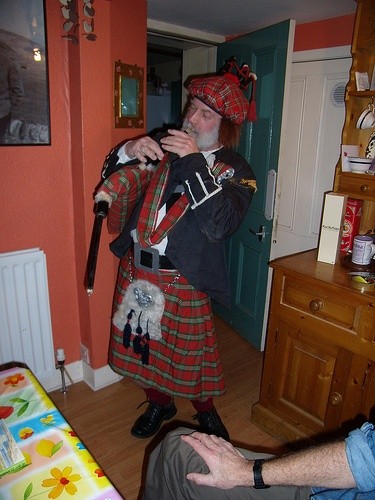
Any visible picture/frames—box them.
[0,0,51,146]
[113,59,145,128]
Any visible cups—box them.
[356,104,375,129]
[352,236,375,266]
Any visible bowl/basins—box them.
[348,157,373,174]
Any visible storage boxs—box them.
[339,197,364,252]
[317,191,347,265]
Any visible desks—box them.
[0,367,124,500]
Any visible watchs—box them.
[253,459,271,489]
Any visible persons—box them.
[102,74,258,442]
[141,422,375,499]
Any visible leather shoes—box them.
[130,401,178,438]
[192,405,231,442]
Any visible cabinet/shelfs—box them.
[252,0,375,448]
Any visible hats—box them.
[187,72,250,124]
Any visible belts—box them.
[130,237,177,275]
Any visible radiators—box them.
[0,246,65,383]
